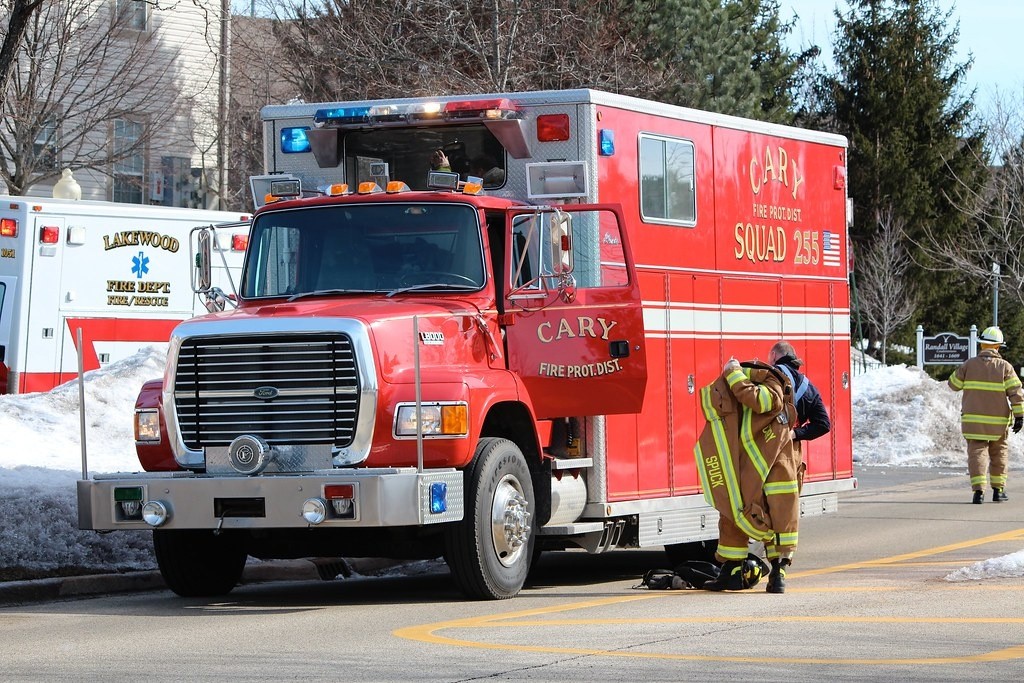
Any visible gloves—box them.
[1012,417,1023,433]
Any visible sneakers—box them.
[766,566,786,593]
[704,561,744,591]
[972,490,984,504]
[992,487,1008,501]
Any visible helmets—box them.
[976,326,1008,347]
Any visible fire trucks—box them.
[77,87,857,600]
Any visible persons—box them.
[428,148,504,189]
[715,342,830,594]
[948,326,1024,504]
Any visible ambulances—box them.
[0,195,262,394]
